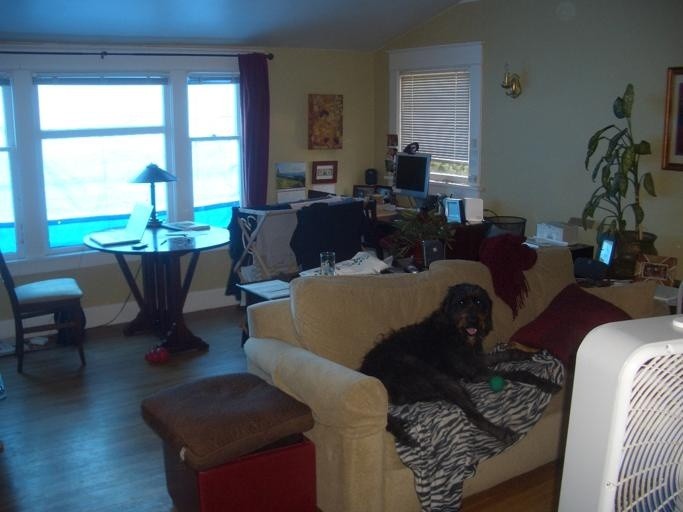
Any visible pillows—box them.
[139,370,319,472]
[508,281,637,375]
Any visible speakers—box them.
[365,169,377,184]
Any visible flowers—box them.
[389,203,453,245]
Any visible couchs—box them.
[242,242,674,510]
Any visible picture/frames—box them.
[310,160,340,186]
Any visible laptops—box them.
[89,202,154,248]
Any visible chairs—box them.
[0,245,84,374]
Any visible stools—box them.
[161,432,315,512]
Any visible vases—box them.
[417,237,434,267]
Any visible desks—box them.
[85,223,231,354]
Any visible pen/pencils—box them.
[160,240,167,246]
[166,234,188,236]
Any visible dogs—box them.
[356,283,563,449]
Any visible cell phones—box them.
[132,242,148,249]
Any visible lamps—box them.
[125,163,179,229]
[494,51,521,100]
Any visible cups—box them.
[320,252,336,277]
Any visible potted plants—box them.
[580,84,662,275]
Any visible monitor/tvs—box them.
[393,152,431,212]
